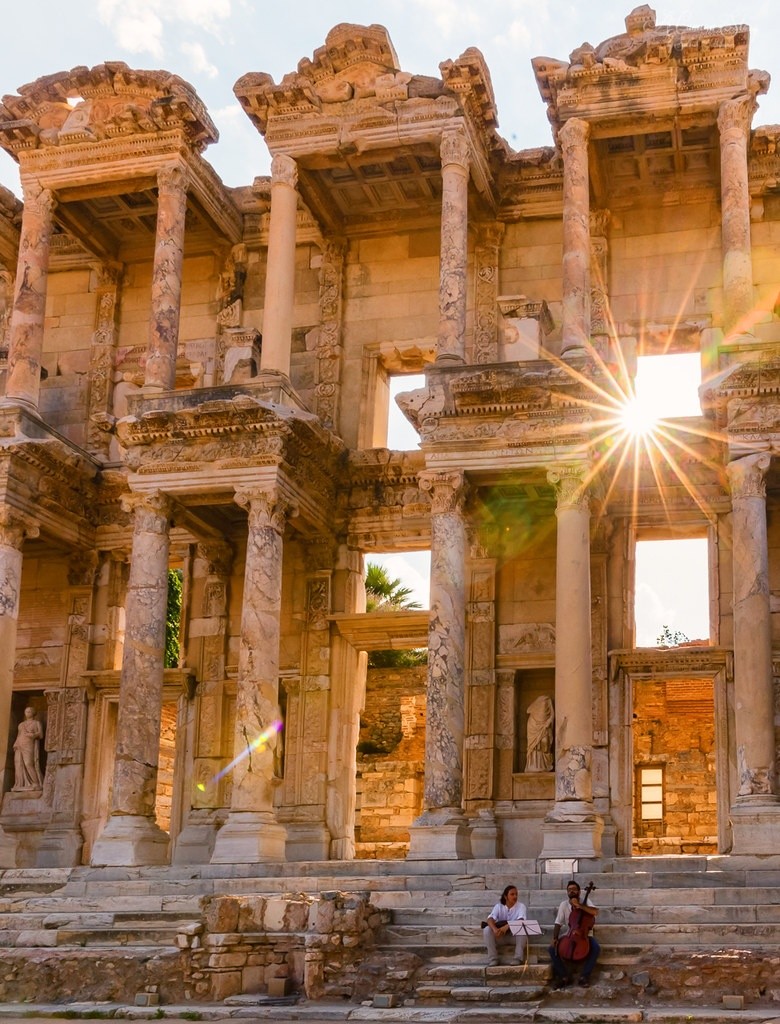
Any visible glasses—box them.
[568,887,578,891]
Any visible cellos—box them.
[558,880,596,991]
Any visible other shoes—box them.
[553,977,571,990]
[510,958,523,966]
[489,958,502,967]
[578,977,589,988]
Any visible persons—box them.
[548,880,600,992]
[10,706,44,791]
[523,695,554,773]
[481,885,527,966]
